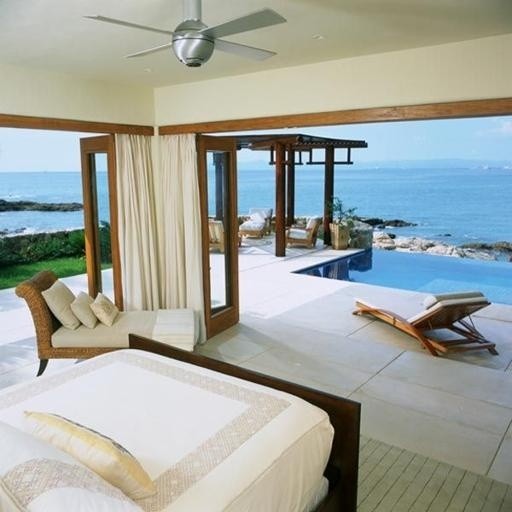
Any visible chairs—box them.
[208,208,322,253]
[352,291,499,357]
[15,270,196,378]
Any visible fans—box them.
[82,0,287,67]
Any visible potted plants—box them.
[324,196,360,251]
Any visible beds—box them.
[2,334,361,511]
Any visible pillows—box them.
[424,291,484,310]
[41,279,119,330]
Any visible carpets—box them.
[356,435,511,511]
